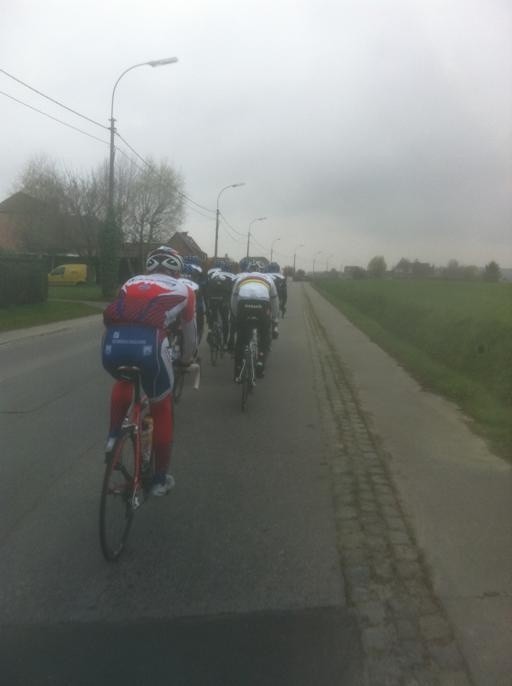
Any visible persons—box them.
[97,243,199,501]
[166,253,291,390]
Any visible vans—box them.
[47,263,96,285]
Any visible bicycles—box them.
[203,294,288,415]
[94,357,203,566]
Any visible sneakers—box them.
[104,435,117,463]
[152,475,175,496]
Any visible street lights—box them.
[313,250,323,273]
[326,253,335,271]
[294,244,306,273]
[246,216,269,258]
[107,56,178,212]
[269,237,281,266]
[214,180,248,259]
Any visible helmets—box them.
[184,255,280,273]
[146,246,184,271]
